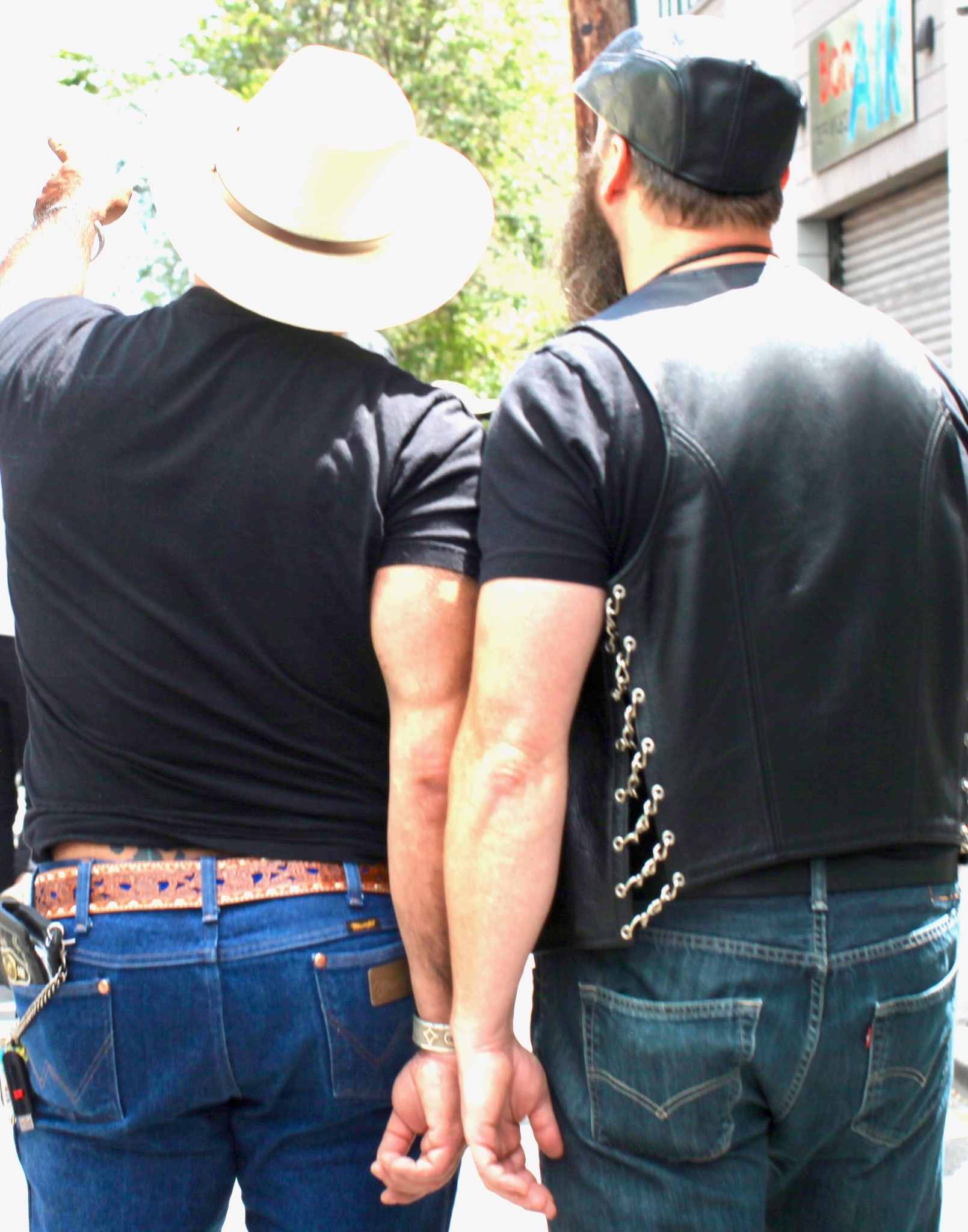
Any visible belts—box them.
[634,847,961,899]
[37,862,395,921]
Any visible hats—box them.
[150,46,494,332]
[571,16,805,194]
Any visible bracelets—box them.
[412,1011,455,1054]
[31,203,107,261]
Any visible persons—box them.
[447,15,968,1231]
[0,43,490,1232]
